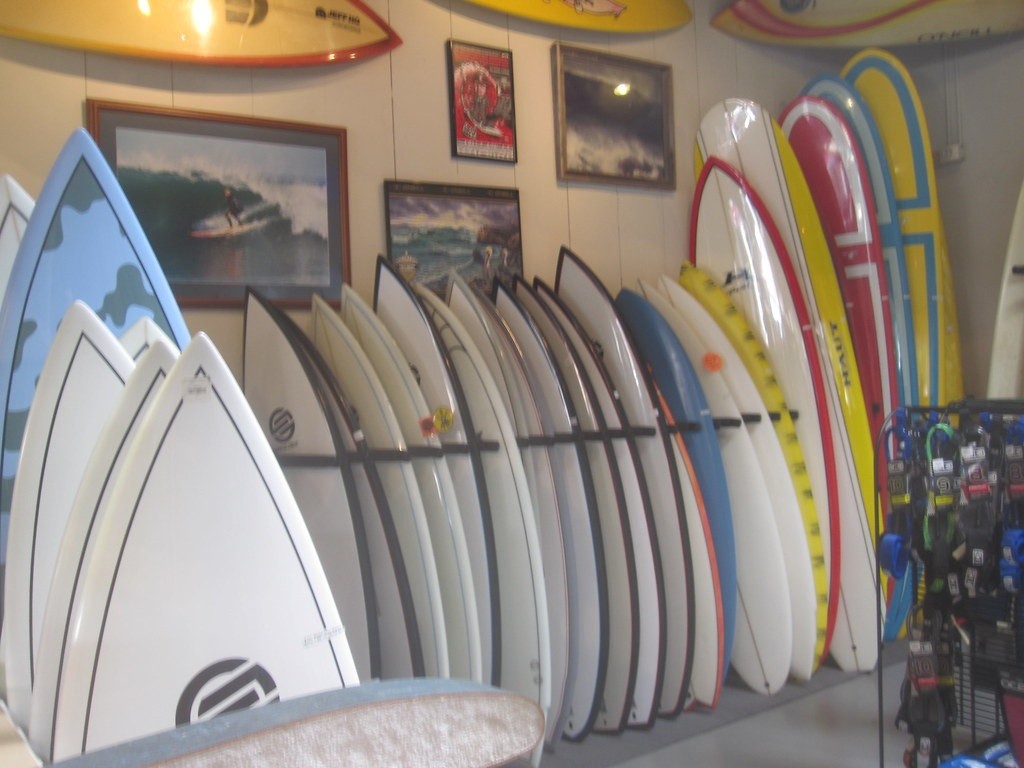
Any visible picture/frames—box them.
[552,41,678,193]
[383,178,523,298]
[446,38,518,164]
[85,98,351,310]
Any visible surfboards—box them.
[0,48,967,767]
[0,0,1024,68]
[986,179,1024,403]
